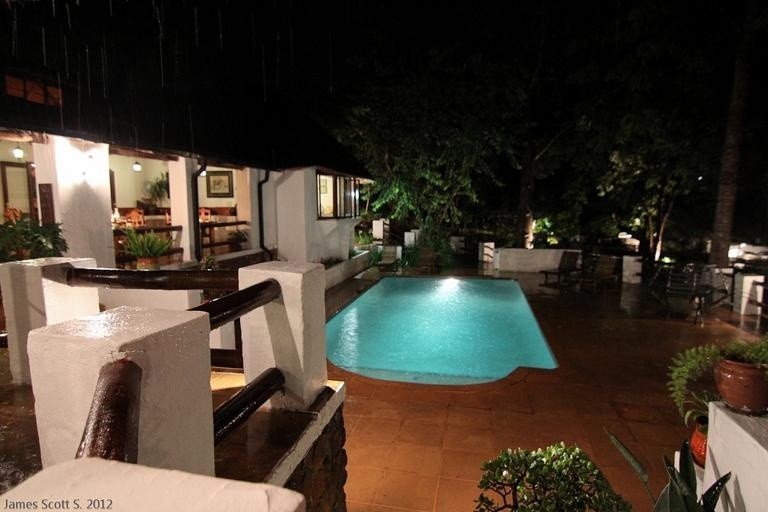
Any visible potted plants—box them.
[116,227,174,268]
[666,336,768,469]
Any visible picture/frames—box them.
[206,171,234,198]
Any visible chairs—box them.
[125,207,211,236]
[538,251,728,324]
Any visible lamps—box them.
[134,156,142,172]
[13,141,22,161]
